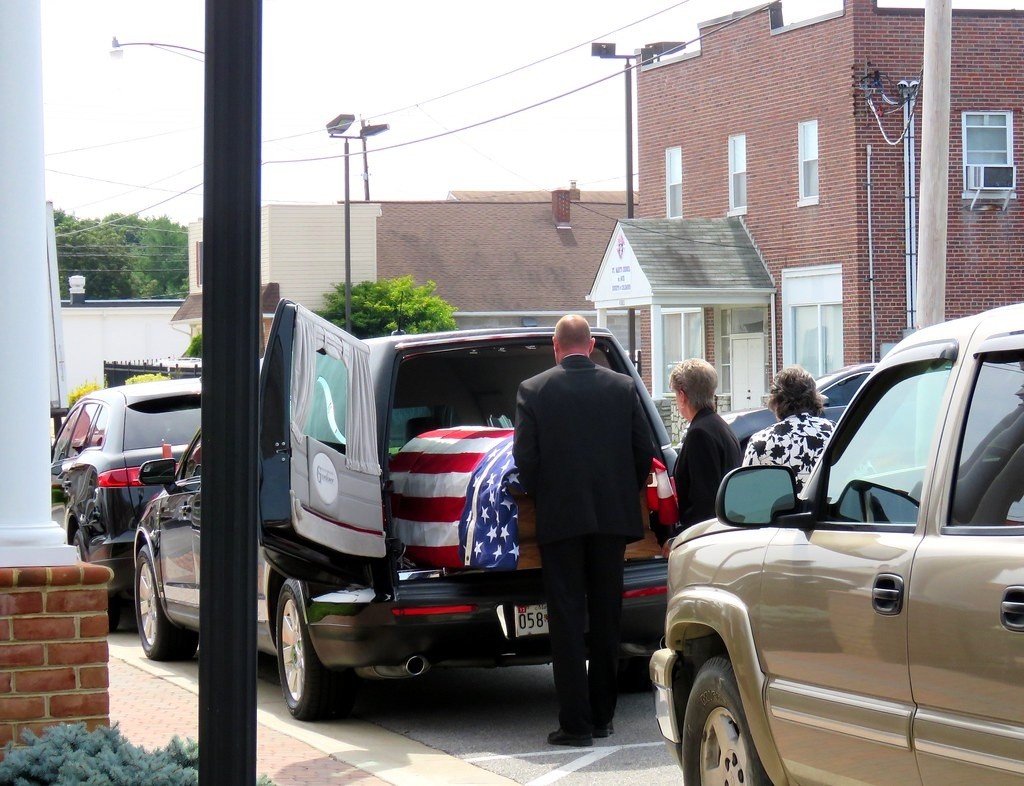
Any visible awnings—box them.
[170,281,280,337]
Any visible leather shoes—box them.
[548,729,593,748]
[592,721,614,738]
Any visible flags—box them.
[390,422,681,573]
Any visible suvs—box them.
[131,300,681,721]
[648,299,1024,786]
[718,364,878,466]
[50,376,202,626]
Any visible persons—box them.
[512,313,658,746]
[661,356,742,562]
[742,363,842,502]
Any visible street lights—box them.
[590,41,640,219]
[110,37,205,62]
[326,114,366,336]
[645,41,686,63]
[360,122,389,201]
